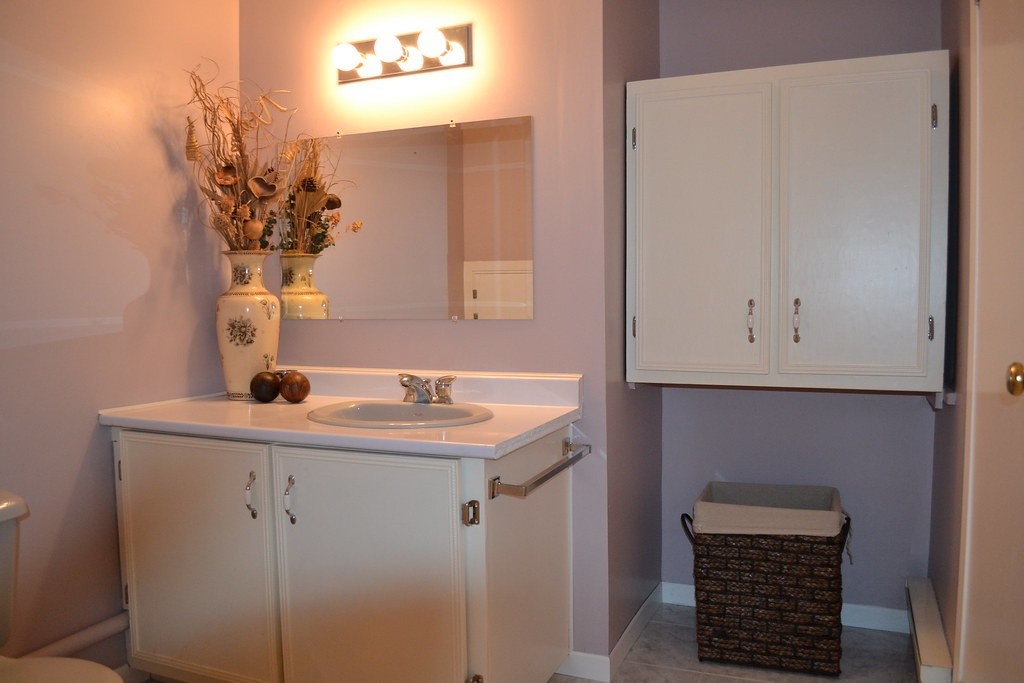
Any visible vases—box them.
[281,249,330,320]
[215,250,281,400]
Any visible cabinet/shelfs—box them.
[110,427,571,683]
[624,47,951,412]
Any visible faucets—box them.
[399,373,458,404]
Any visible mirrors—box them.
[276,114,535,321]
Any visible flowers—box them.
[176,52,311,252]
[278,139,365,252]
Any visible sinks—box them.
[306,399,495,429]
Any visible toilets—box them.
[0,489,125,683]
